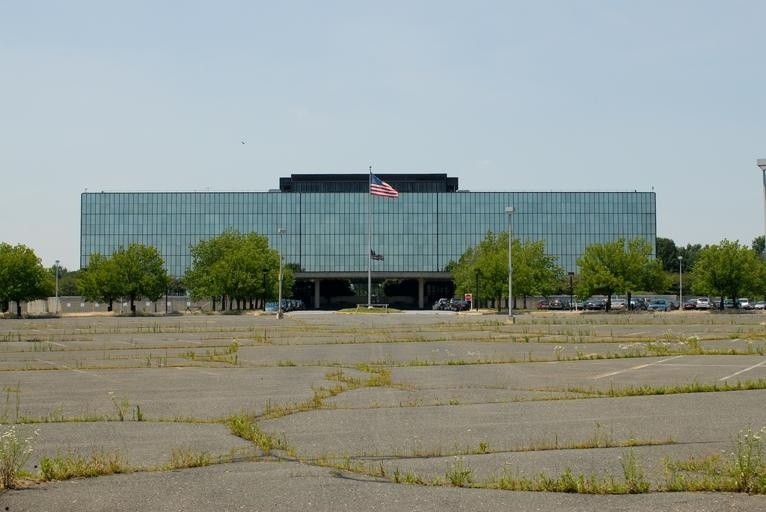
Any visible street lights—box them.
[757,159,766,253]
[474,268,481,311]
[277,229,286,319]
[56,260,60,313]
[568,272,574,311]
[677,256,683,310]
[505,206,514,317]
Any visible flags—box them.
[369,173,399,198]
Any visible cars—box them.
[537,297,766,311]
[432,298,469,311]
[265,299,304,312]
